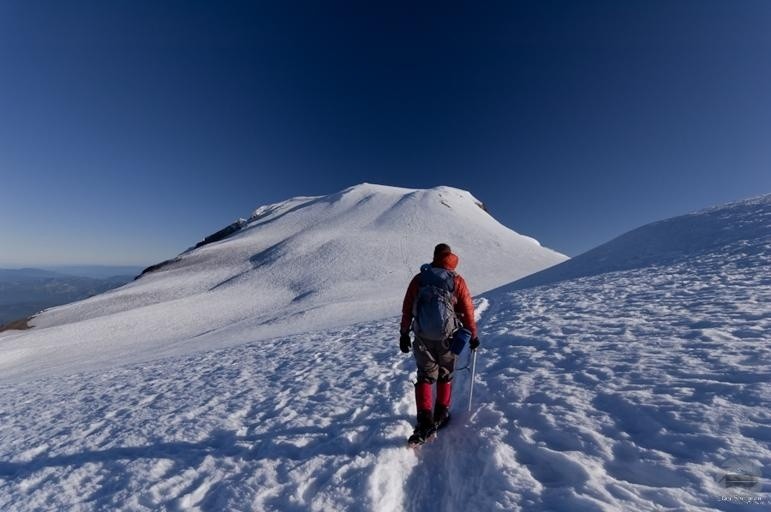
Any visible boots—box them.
[408,412,451,447]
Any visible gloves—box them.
[399,328,412,353]
[469,337,480,349]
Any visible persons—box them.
[400,242,481,444]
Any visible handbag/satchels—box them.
[448,327,472,355]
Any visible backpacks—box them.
[412,263,455,341]
